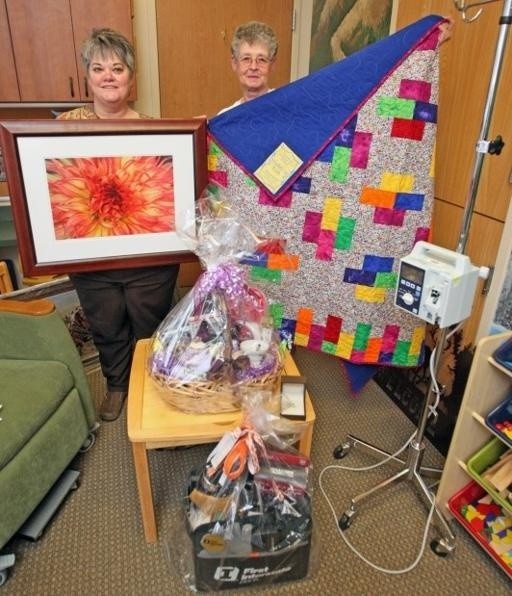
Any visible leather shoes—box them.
[99,392,126,421]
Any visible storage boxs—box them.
[187,483,313,596]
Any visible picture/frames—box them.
[0,275,103,375]
[290,0,400,92]
[0,116,217,281]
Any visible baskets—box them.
[149,289,284,412]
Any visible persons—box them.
[54,27,180,420]
[216,21,296,355]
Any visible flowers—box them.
[45,154,178,240]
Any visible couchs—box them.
[0,294,103,581]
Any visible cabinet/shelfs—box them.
[1,0,20,103]
[432,197,510,401]
[428,328,512,588]
[399,1,511,221]
[7,0,137,103]
[154,1,294,116]
